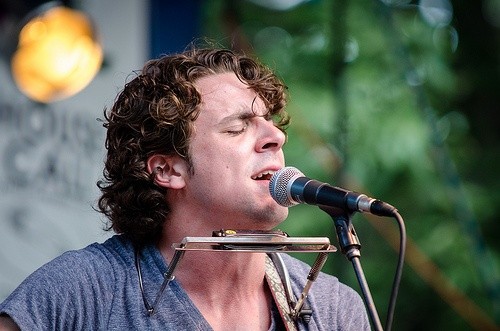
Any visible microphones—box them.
[269,166,399,218]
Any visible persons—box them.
[0,35,373,331]
